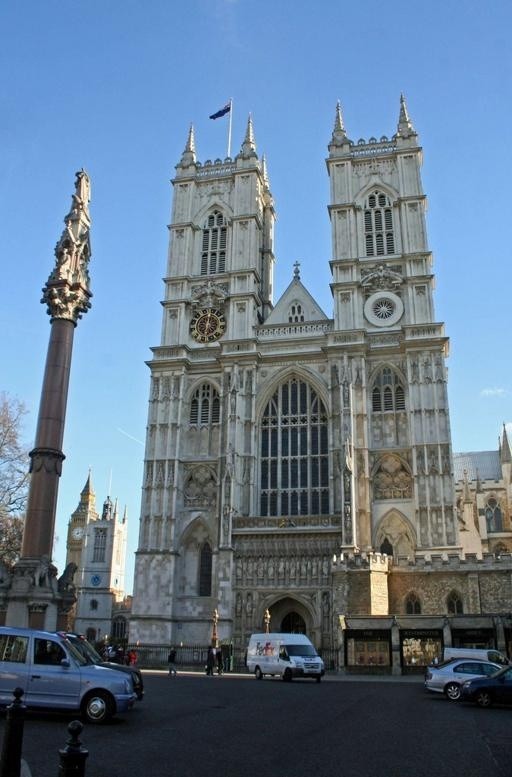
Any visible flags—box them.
[210,96,233,121]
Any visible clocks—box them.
[70,527,85,541]
[190,307,229,344]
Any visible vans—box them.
[246,632,325,682]
[443,646,512,667]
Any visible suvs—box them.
[51,629,145,698]
[0,625,138,719]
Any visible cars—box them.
[425,657,511,699]
[460,664,511,706]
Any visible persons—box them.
[206,646,215,675]
[129,648,140,665]
[216,646,225,676]
[168,649,178,677]
[212,646,217,660]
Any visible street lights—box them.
[264,607,271,633]
[211,608,220,648]
